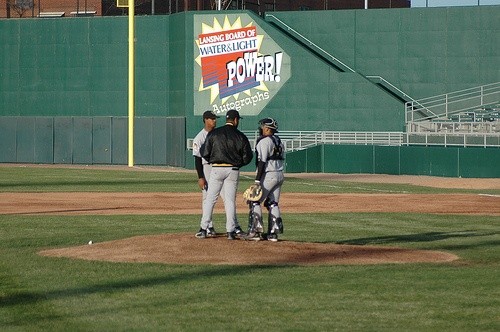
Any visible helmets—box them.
[258,118,279,133]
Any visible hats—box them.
[226,109,243,119]
[203,110,220,120]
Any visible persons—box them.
[195,108,253,240]
[192,110,242,236]
[243,117,284,242]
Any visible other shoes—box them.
[206,227,217,238]
[234,225,246,237]
[264,234,278,242]
[194,228,206,239]
[226,232,235,240]
[245,232,264,241]
